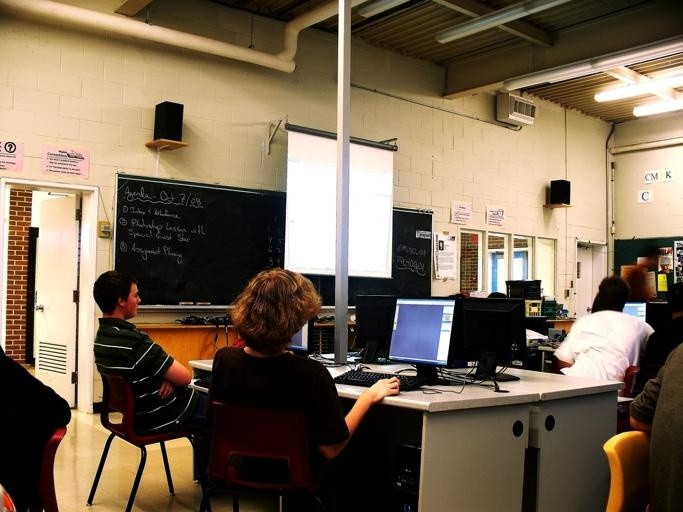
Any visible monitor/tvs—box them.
[622,301,649,323]
[649,301,672,330]
[461,298,527,382]
[356,295,400,365]
[387,297,461,385]
[285,319,314,358]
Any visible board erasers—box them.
[196,303,212,306]
[179,301,194,306]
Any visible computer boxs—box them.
[376,442,421,512]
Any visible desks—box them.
[185,354,538,512]
[325,352,625,512]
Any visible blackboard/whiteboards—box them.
[112,173,434,312]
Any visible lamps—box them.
[356,0,407,18]
[436,0,569,44]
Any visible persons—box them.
[636,282,683,394]
[92,270,208,471]
[1,347,73,511]
[207,266,402,511]
[554,274,652,385]
[624,245,663,302]
[658,256,674,274]
[628,339,682,511]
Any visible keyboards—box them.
[347,351,360,356]
[334,369,427,391]
[194,376,212,389]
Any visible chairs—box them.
[601,428,648,512]
[87,368,198,512]
[27,427,65,512]
[202,402,328,511]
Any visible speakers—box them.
[154,101,183,141]
[550,180,570,204]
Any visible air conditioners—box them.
[495,92,536,126]
